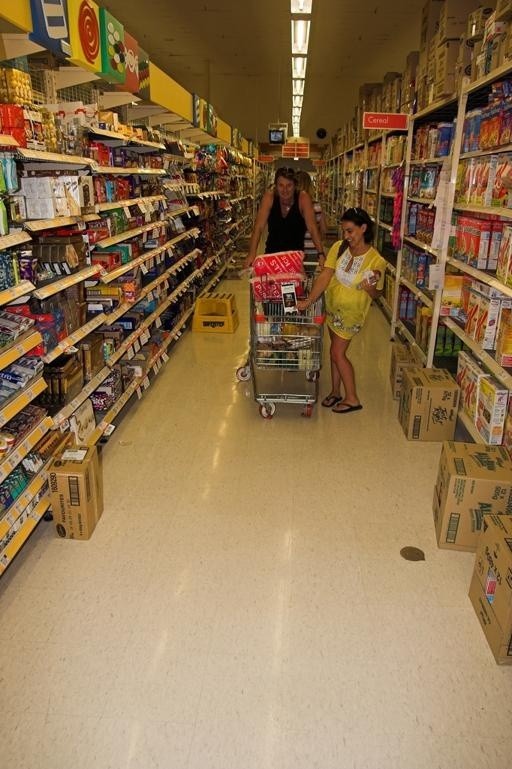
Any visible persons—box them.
[245,167,329,271]
[296,171,326,278]
[294,206,387,412]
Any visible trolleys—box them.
[232,259,325,419]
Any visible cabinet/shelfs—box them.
[1,11,286,574]
[306,12,512,443]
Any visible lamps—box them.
[290,0,311,13]
[288,13,310,161]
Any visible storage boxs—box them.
[469,515,512,667]
[437,0,481,46]
[420,0,443,53]
[432,443,510,555]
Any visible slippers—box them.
[332,402,362,413]
[321,395,342,407]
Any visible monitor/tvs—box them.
[269,130,285,145]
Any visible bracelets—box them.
[305,298,313,306]
[317,252,324,258]
